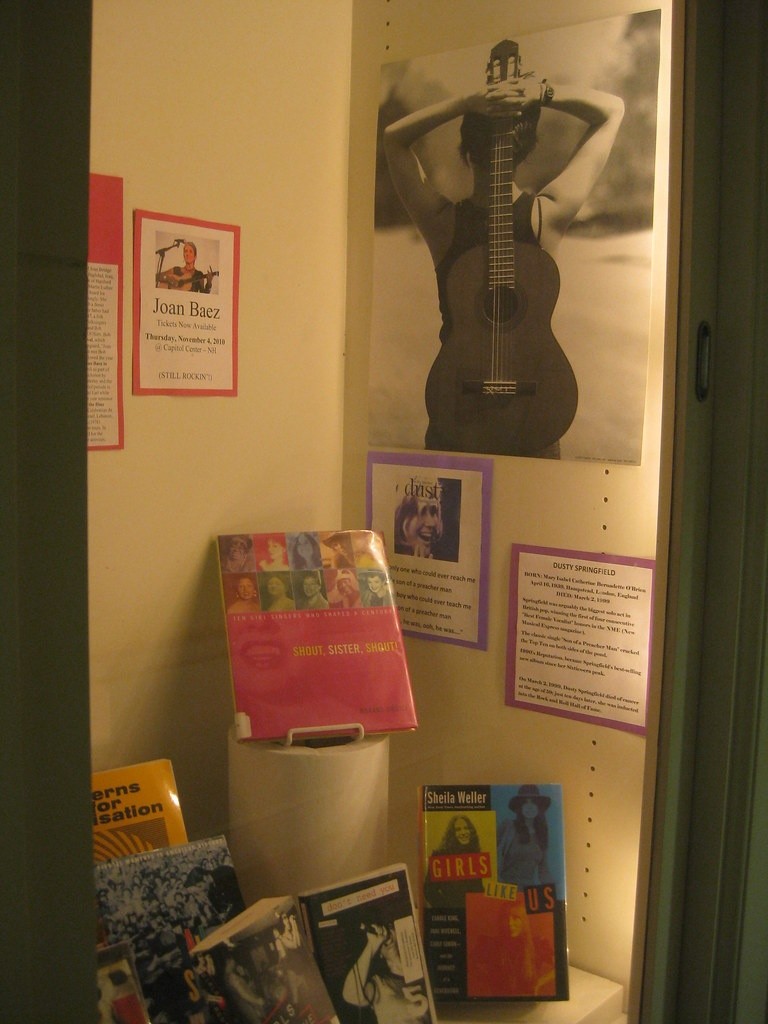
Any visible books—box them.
[95,940,152,1024]
[414,781,570,1004]
[92,834,248,1024]
[295,863,437,1023]
[90,757,189,869]
[189,895,342,1023]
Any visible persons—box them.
[222,953,269,1023]
[191,950,215,979]
[338,916,432,1024]
[422,810,485,910]
[92,834,247,1024]
[383,72,624,463]
[469,898,555,997]
[392,486,447,562]
[495,784,552,894]
[219,528,398,615]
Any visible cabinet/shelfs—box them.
[434,965,626,1022]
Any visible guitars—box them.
[424,39,577,454]
[158,270,218,290]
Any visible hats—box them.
[323,531,349,546]
[509,784,551,813]
[357,569,387,581]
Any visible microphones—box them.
[175,239,187,244]
[361,924,378,935]
